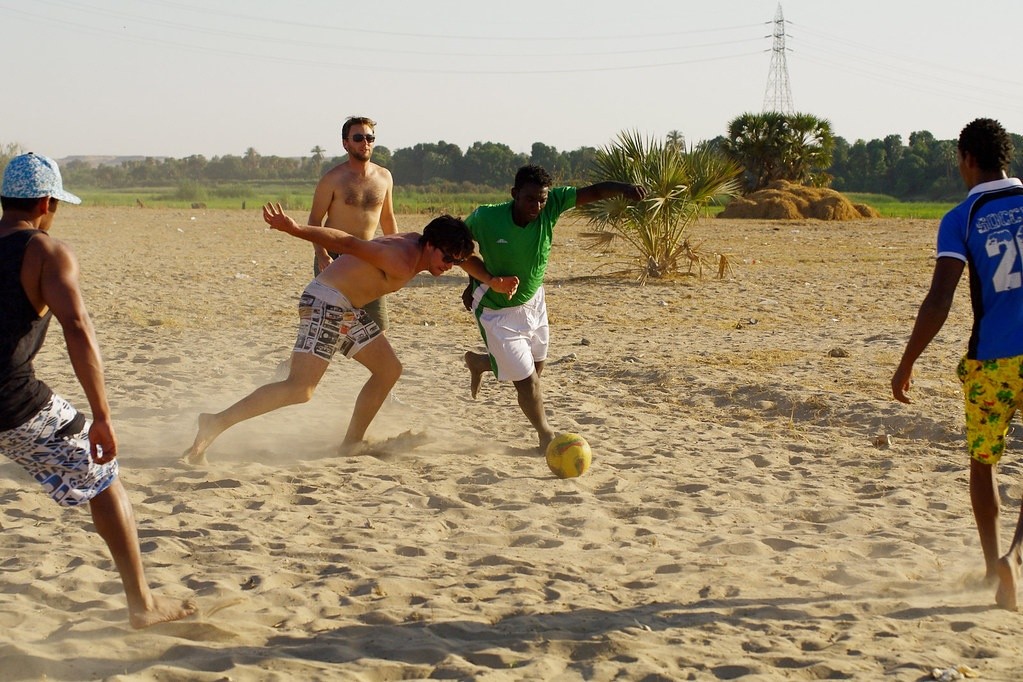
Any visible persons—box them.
[0,152,194,628]
[891,117,1023,612]
[277,116,411,407]
[182,203,475,467]
[454,164,648,448]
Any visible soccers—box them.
[545,432,592,478]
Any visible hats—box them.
[2,152,82,205]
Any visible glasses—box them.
[346,134,375,142]
[440,248,464,265]
